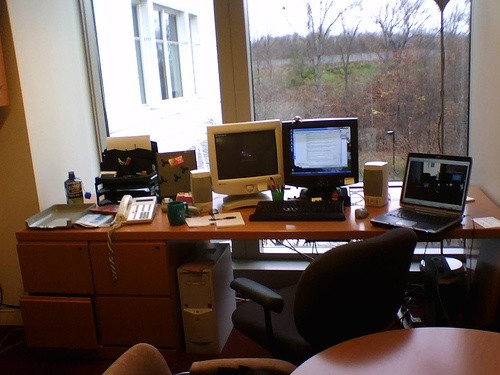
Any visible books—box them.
[74,213,114,229]
[105,134,152,150]
[465,195,475,203]
[185,215,213,227]
[213,212,246,227]
[473,216,500,230]
[157,149,198,196]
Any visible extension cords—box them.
[401,308,421,326]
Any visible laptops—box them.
[370,152,472,234]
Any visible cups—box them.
[168,201,189,226]
[272,191,283,201]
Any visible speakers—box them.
[363,161,389,207]
[190,169,212,212]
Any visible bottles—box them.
[64,171,84,204]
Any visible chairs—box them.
[229,228,417,361]
[102,343,297,374]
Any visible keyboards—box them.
[249,201,346,222]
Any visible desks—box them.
[16,184,499,360]
[290,326,500,374]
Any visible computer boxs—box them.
[177,243,236,354]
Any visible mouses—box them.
[355,208,369,220]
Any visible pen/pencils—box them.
[209,217,235,220]
[268,176,284,192]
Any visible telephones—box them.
[107,195,156,280]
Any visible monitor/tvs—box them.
[207,119,284,211]
[282,117,358,206]
